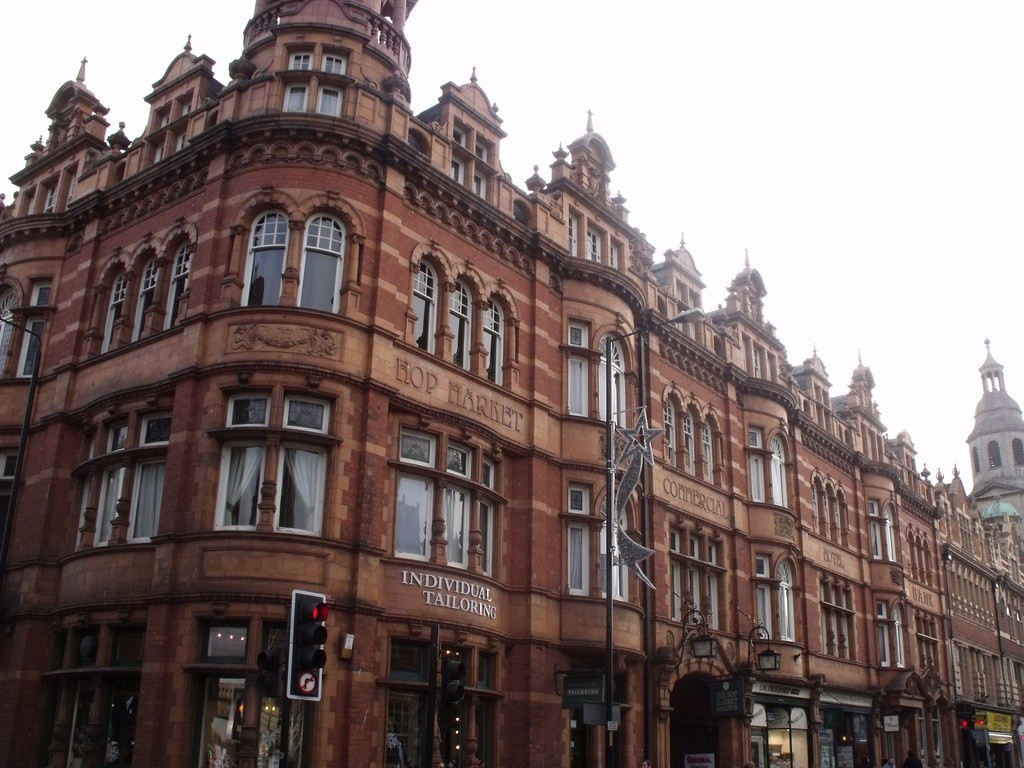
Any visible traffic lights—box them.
[286,590,328,701]
[958,702,971,729]
[441,658,465,705]
[255,650,284,696]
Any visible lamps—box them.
[745,620,781,682]
[675,603,717,673]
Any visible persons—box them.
[883,757,895,768]
[902,750,923,768]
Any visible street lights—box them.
[606,307,707,768]
